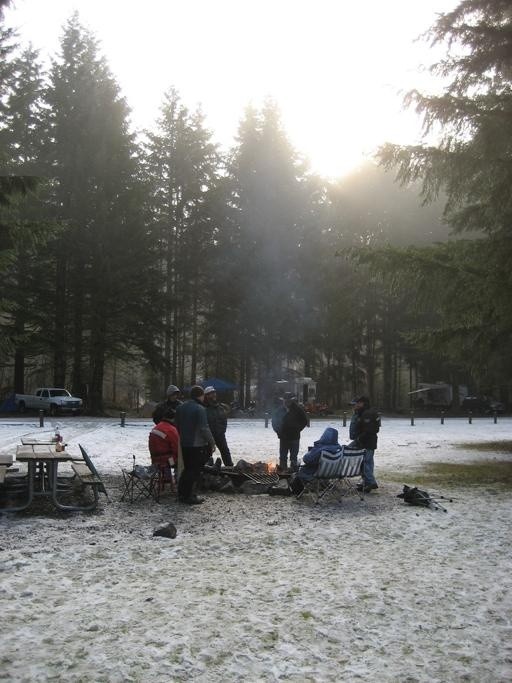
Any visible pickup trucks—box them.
[14,387,83,418]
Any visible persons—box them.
[354,393,382,491]
[294,426,341,479]
[270,388,309,469]
[164,384,185,425]
[349,395,360,439]
[174,384,216,504]
[204,384,234,466]
[150,411,222,472]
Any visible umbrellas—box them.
[190,377,241,396]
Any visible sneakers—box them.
[356,483,378,493]
[178,495,201,504]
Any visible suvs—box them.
[464,397,505,417]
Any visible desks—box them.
[15,436,72,514]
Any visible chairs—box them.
[298,446,368,505]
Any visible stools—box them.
[121,464,164,504]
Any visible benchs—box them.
[0,455,101,512]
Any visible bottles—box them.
[56,441,62,452]
[55,427,61,443]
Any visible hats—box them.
[278,392,296,401]
[204,386,216,395]
[348,395,362,406]
[163,408,177,423]
[191,386,204,398]
[166,385,180,396]
